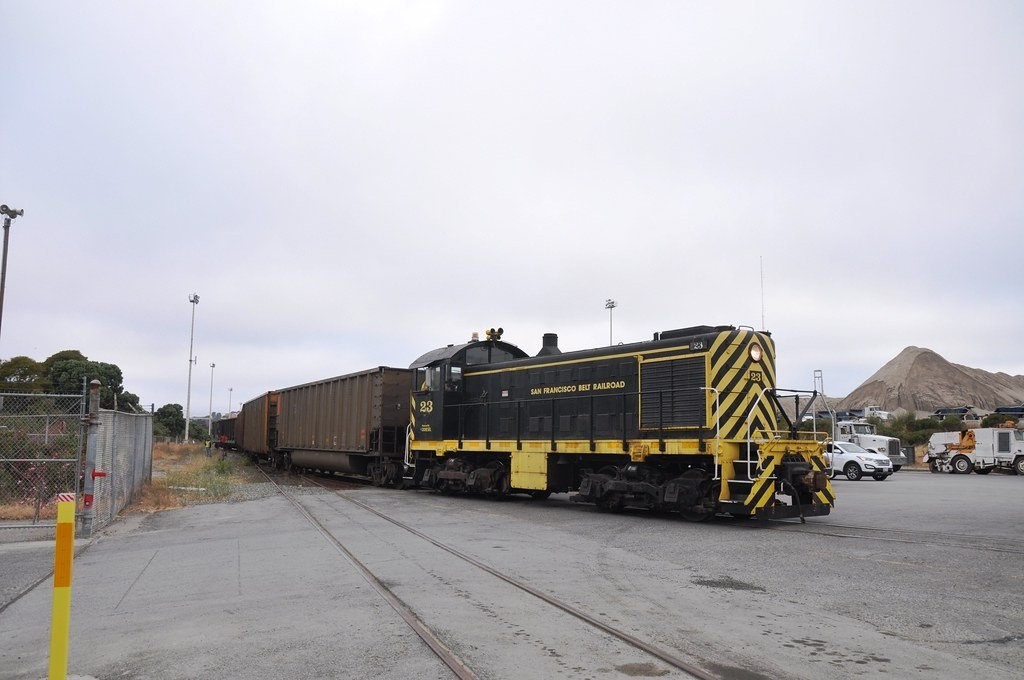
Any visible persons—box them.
[220,433,228,456]
[205,437,213,457]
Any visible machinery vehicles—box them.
[923,427,1024,475]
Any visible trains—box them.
[210,324,834,525]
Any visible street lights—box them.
[605,299,618,346]
[229,387,233,415]
[180,290,202,442]
[210,363,216,415]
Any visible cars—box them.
[802,412,813,422]
[816,440,894,482]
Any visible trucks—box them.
[832,419,907,472]
[816,406,893,422]
